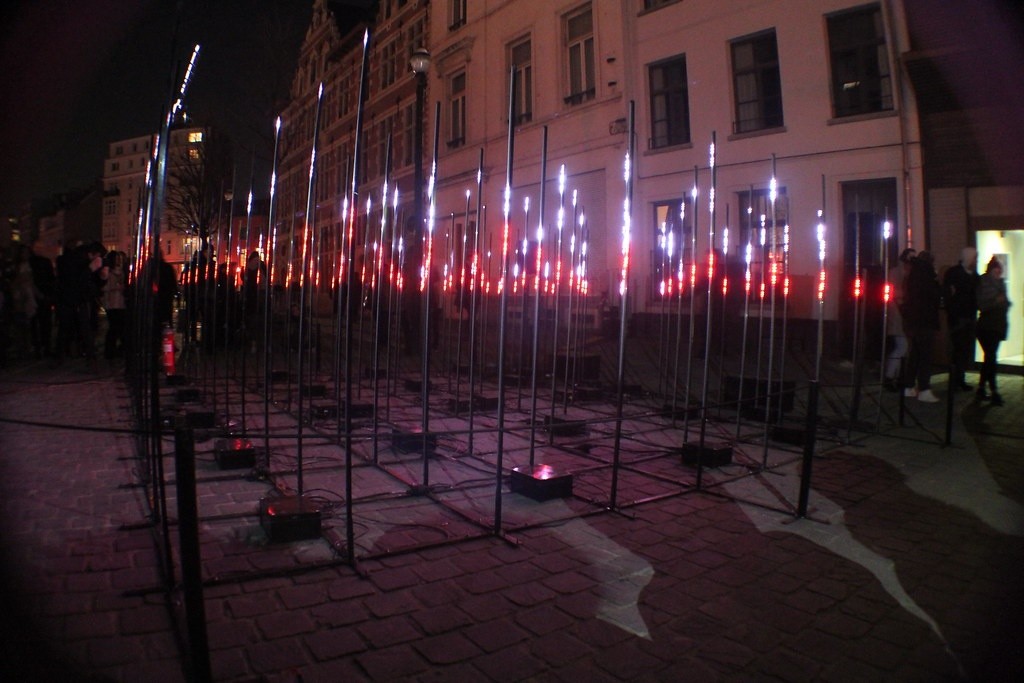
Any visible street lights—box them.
[408,45,430,360]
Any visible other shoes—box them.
[904,387,917,397]
[955,382,969,390]
[918,390,939,403]
[990,392,1003,402]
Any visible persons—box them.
[0,242,442,375]
[976,256,1012,405]
[885,248,942,402]
[943,247,980,393]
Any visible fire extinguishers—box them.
[161,322,176,377]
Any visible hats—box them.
[988,257,1002,271]
